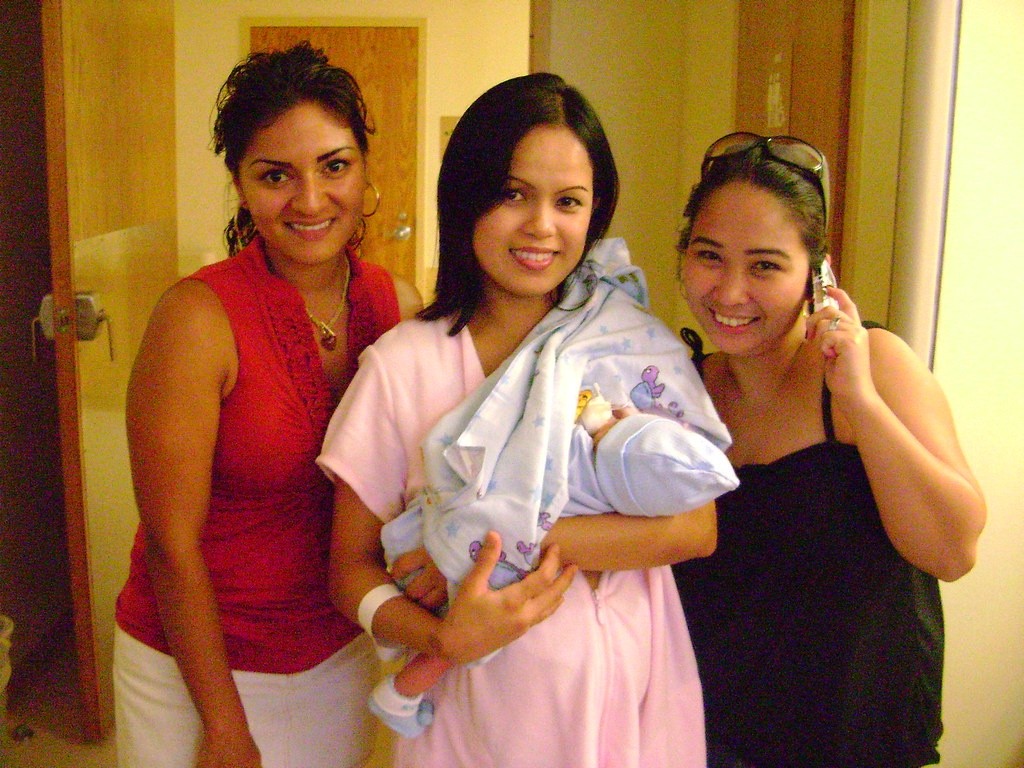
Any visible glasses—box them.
[701,131,828,234]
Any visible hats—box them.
[594,414,741,518]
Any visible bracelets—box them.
[357,580,404,637]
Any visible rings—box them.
[826,317,839,332]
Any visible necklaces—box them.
[306,251,350,350]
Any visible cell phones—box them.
[811,262,839,315]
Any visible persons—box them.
[368,402,742,738]
[672,131,986,768]
[315,73,742,768]
[111,38,424,768]
